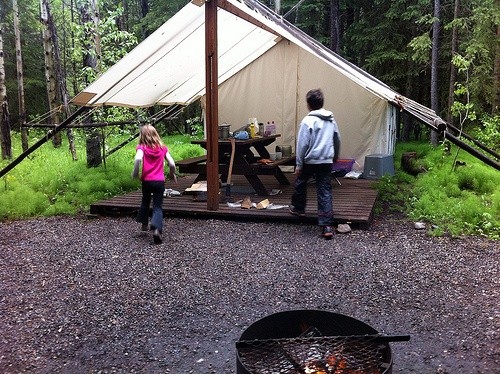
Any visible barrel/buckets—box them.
[218,125,230,138]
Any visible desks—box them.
[181,134,291,196]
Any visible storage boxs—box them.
[362,154,394,179]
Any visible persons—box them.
[131,125,177,245]
[290,88,342,237]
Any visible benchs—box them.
[167,155,206,195]
[250,154,296,196]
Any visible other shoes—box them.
[321,226,334,237]
[219,175,228,186]
[153,229,163,244]
[289,204,306,216]
[141,224,148,231]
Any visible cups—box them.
[269,153,278,160]
[277,152,282,160]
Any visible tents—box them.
[0,0,500,179]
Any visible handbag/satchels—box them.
[276,145,292,157]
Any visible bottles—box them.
[250,123,254,138]
[266,121,271,136]
[271,121,276,134]
[259,123,264,135]
